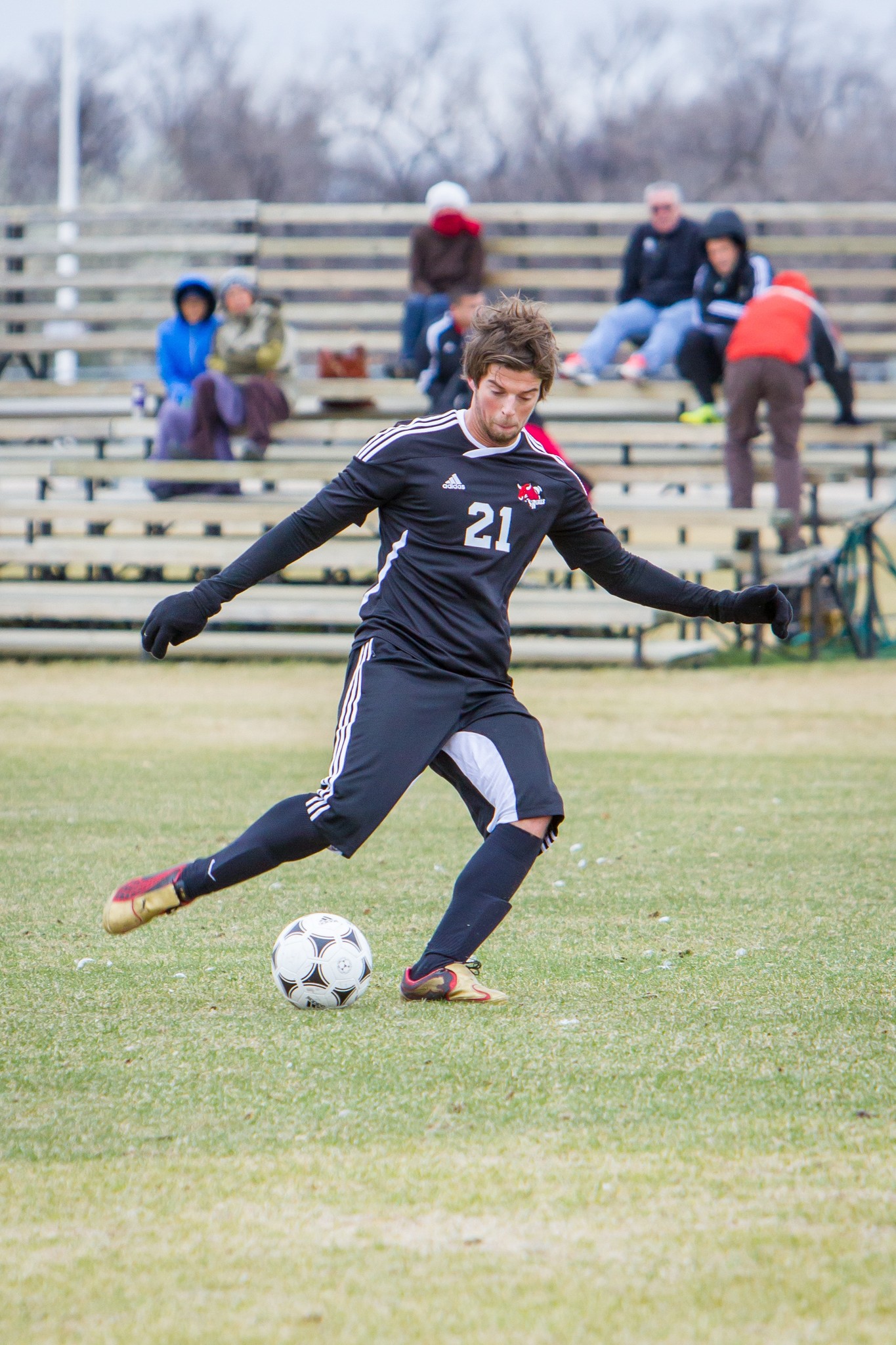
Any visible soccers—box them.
[272,912,374,1012]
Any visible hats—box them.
[217,264,260,301]
[425,180,471,219]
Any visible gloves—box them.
[725,582,794,640]
[140,591,209,660]
[833,406,861,426]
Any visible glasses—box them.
[652,204,672,215]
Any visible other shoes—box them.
[617,354,651,388]
[777,536,807,555]
[557,353,598,388]
[678,403,725,423]
[735,529,758,550]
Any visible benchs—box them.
[2,379,894,674]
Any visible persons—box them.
[174,272,294,460]
[389,180,490,378]
[141,272,226,499]
[414,281,495,423]
[717,267,860,556]
[674,204,776,425]
[98,288,797,1008]
[555,178,709,388]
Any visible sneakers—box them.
[103,863,195,935]
[400,962,508,1003]
